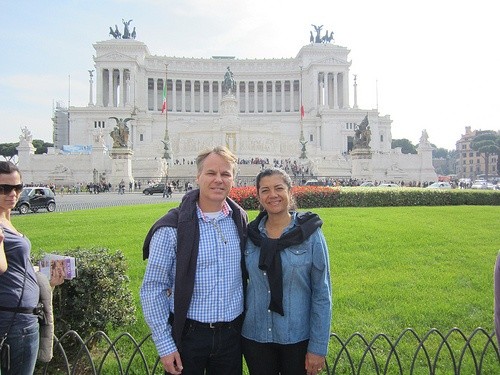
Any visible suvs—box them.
[11,187,56,214]
[143,183,174,195]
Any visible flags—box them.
[162,73,167,114]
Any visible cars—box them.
[361,182,399,187]
[428,179,500,190]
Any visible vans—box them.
[305,181,325,186]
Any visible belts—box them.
[0,306,33,313]
[185,319,241,331]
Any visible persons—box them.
[244,167,331,375]
[23,155,472,197]
[110,118,128,148]
[354,114,372,149]
[494,251,500,338]
[140,146,247,375]
[0,161,66,375]
[224,66,236,95]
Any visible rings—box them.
[318,368,322,373]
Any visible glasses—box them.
[0,184,23,194]
